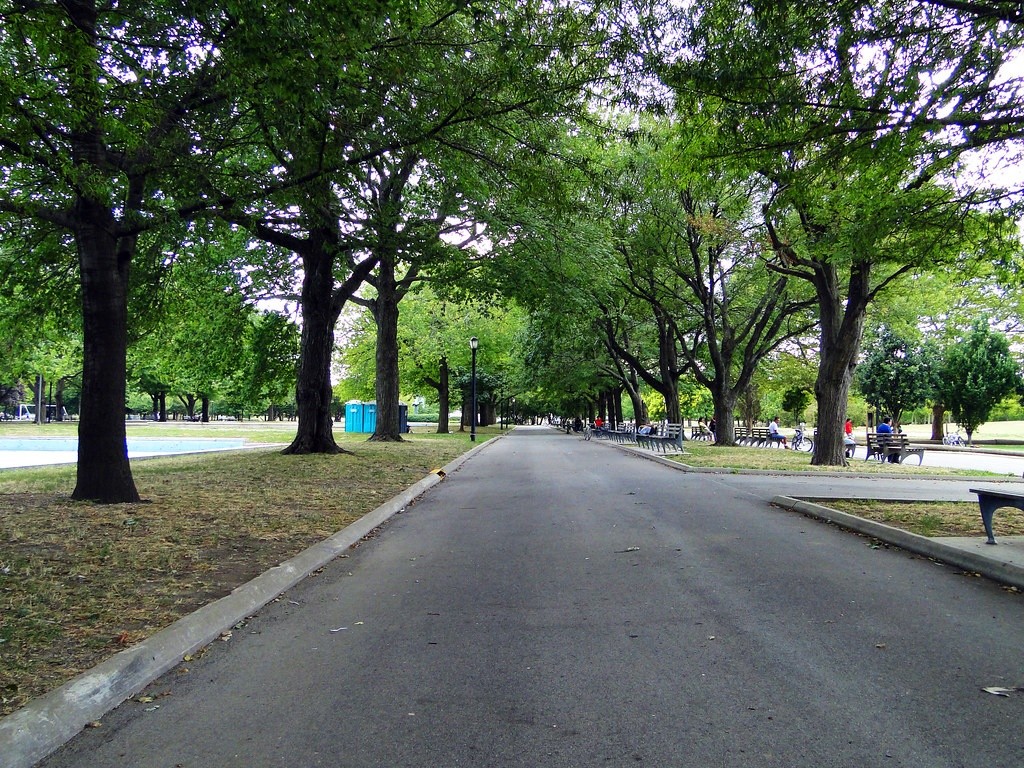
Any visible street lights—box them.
[470,337,479,441]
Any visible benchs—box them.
[691,426,712,440]
[591,423,612,437]
[635,422,685,454]
[732,427,752,446]
[866,433,927,465]
[969,488,1024,544]
[183,416,200,422]
[608,422,636,443]
[750,427,781,448]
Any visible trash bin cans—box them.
[398,400,408,433]
[363,400,377,432]
[344,399,364,434]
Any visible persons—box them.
[845,418,853,437]
[575,417,582,432]
[769,417,790,449]
[877,418,900,463]
[595,416,604,436]
[698,417,717,441]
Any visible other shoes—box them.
[785,445,790,449]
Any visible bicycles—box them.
[790,423,814,452]
[584,424,592,440]
[942,429,968,447]
